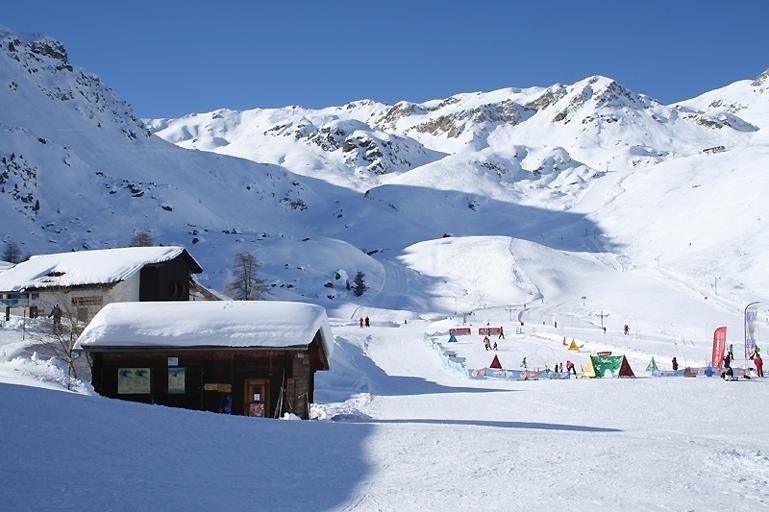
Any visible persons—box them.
[754,354,763,378]
[483,336,492,351]
[671,357,678,376]
[358,317,363,327]
[493,342,497,351]
[520,357,527,368]
[498,326,505,339]
[365,315,370,327]
[722,352,731,368]
[558,363,563,372]
[555,364,559,372]
[48,303,63,335]
[566,360,577,378]
[728,343,734,360]
